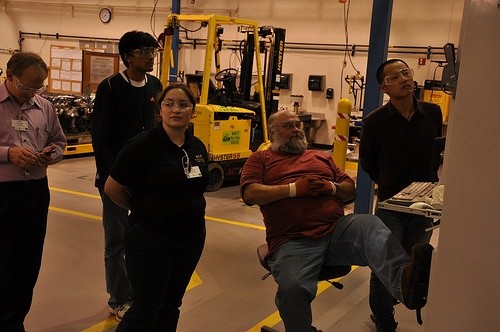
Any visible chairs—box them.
[258,244,351,332]
[185,73,239,107]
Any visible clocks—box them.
[99,8,111,23]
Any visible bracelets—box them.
[289,183,296,196]
[329,181,339,195]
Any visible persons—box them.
[89,30,164,322]
[0,51,68,332]
[359,58,443,332]
[104,82,209,332]
[240,110,434,332]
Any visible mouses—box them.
[410,202,435,210]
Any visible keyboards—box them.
[392,181,438,201]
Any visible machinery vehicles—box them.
[155,11,314,193]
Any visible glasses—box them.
[381,67,414,87]
[160,100,193,112]
[128,46,158,59]
[14,74,46,96]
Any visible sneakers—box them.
[109,304,131,321]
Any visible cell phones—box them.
[45,150,57,155]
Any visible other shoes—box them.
[402,242,433,310]
